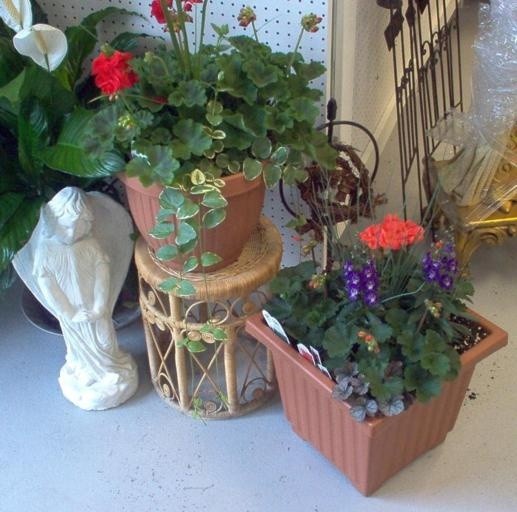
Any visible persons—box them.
[31,186,133,386]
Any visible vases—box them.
[10,186,138,414]
[246,295,508,496]
[117,162,269,275]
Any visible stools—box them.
[130,218,283,420]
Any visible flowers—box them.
[0,0,122,287]
[255,155,492,420]
[88,0,330,182]
